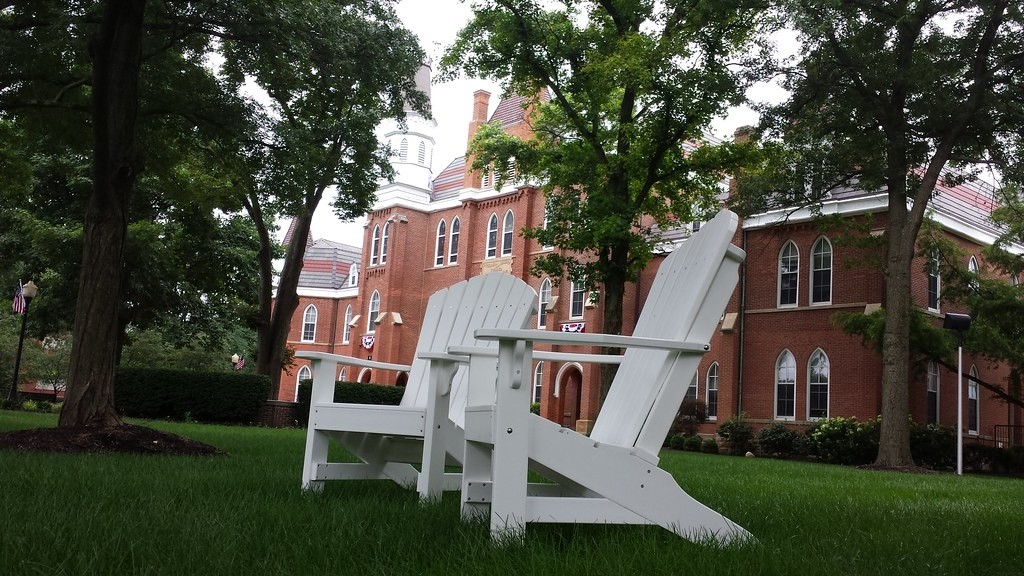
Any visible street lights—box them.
[231,353,239,370]
[9,280,39,411]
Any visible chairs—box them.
[448,208,767,553]
[291,271,539,510]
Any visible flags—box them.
[236,353,245,369]
[12,281,26,314]
